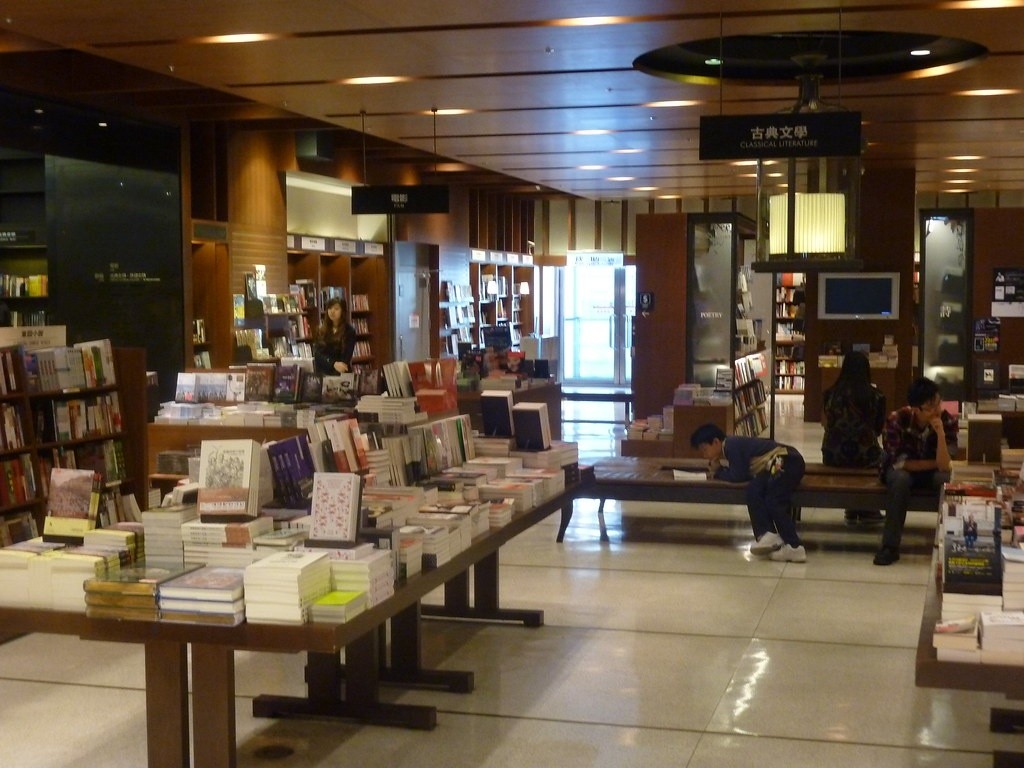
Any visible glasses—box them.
[922,398,941,412]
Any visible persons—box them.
[312,297,357,376]
[873,377,958,565]
[691,423,807,562]
[819,350,885,524]
[963,514,977,547]
[932,448,1024,666]
[996,272,1004,282]
[793,286,805,331]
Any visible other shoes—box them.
[844,509,857,523]
[873,545,899,564]
[858,511,884,523]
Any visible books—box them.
[626,368,733,440]
[962,361,1024,420]
[232,279,371,375]
[445,275,522,354]
[775,287,805,390]
[974,317,1001,352]
[0,274,142,548]
[735,272,769,436]
[818,334,898,368]
[0,359,580,627]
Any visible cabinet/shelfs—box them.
[0,236,1024,645]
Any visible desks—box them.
[913,484,1024,693]
[0,464,594,768]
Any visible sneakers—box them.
[750,531,783,553]
[770,545,807,563]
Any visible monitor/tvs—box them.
[816,270,900,321]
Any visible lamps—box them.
[751,86,864,275]
[526,240,535,255]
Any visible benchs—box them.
[553,456,939,544]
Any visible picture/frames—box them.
[817,272,900,319]
